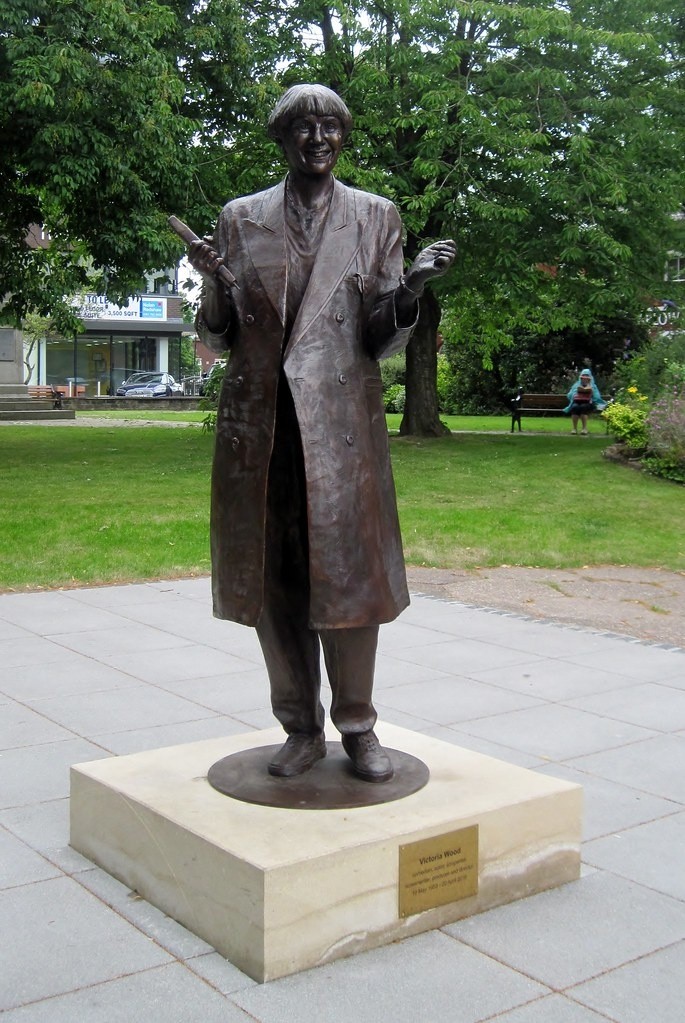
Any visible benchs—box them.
[27,385,63,399]
[510,387,616,435]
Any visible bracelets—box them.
[399,275,425,297]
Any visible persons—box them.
[189,83,458,782]
[564,368,605,435]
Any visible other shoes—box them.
[570,428,577,435]
[580,428,589,435]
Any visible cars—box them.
[199,364,227,397]
[177,376,202,391]
[116,372,183,397]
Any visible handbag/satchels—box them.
[596,403,606,410]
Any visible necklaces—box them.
[285,175,333,229]
